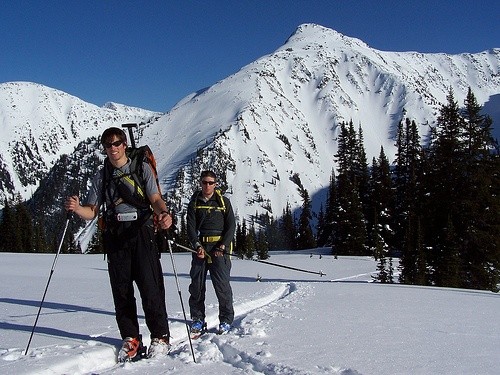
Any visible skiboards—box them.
[174,329,208,350]
[92,353,149,375]
[147,347,177,361]
[218,325,234,336]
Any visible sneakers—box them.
[190,320,207,333]
[148,337,171,356]
[219,323,232,335]
[118,335,146,362]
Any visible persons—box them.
[187,171,236,336]
[65,127,173,364]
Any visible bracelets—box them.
[160,211,168,215]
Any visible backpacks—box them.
[99,145,161,209]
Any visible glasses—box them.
[201,180,215,185]
[104,140,122,148]
